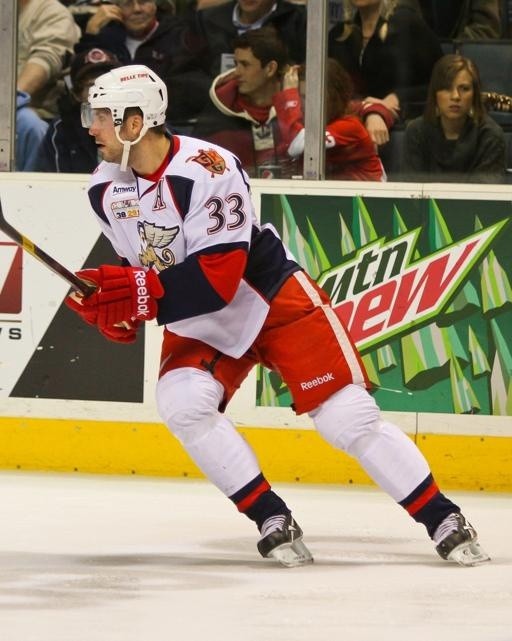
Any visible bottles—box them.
[70,47,121,85]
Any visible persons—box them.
[189,27,397,181]
[390,1,502,129]
[64,63,478,560]
[172,1,306,112]
[328,0,395,97]
[378,54,508,181]
[14,1,81,172]
[272,58,388,181]
[46,1,191,173]
[64,63,492,569]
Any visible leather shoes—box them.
[63,282,140,343]
[74,263,165,330]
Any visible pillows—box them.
[80,64,169,146]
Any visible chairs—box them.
[432,512,477,560]
[257,512,304,558]
